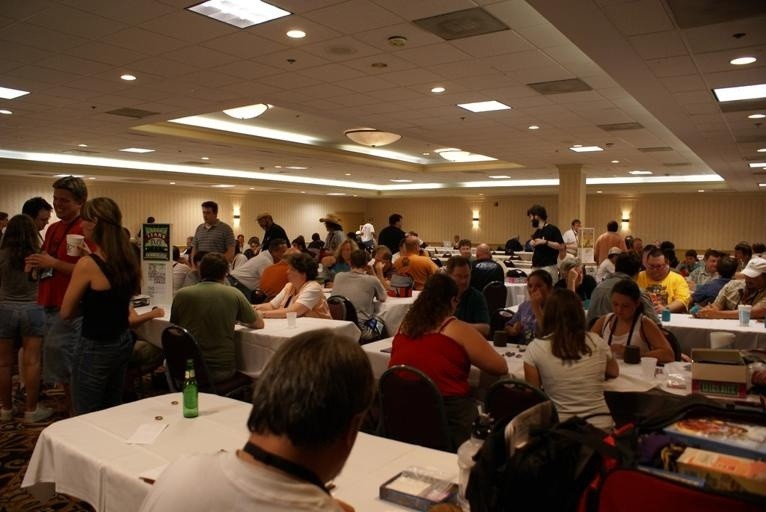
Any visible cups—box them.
[286,311,297,330]
[623,344,641,365]
[66,234,84,257]
[662,309,671,322]
[641,357,657,381]
[494,331,507,346]
[739,304,753,326]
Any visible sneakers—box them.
[23,403,54,423]
[0,404,18,421]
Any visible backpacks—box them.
[464,377,624,511]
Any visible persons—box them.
[0,175,765,456]
[133,327,375,511]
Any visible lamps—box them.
[223,102,270,122]
[343,126,403,149]
[433,147,472,162]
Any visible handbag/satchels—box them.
[387,271,413,298]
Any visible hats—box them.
[608,246,623,255]
[740,257,766,278]
[254,211,271,221]
[320,212,344,231]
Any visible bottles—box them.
[457,421,489,512]
[182,357,200,417]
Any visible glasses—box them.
[450,272,470,279]
